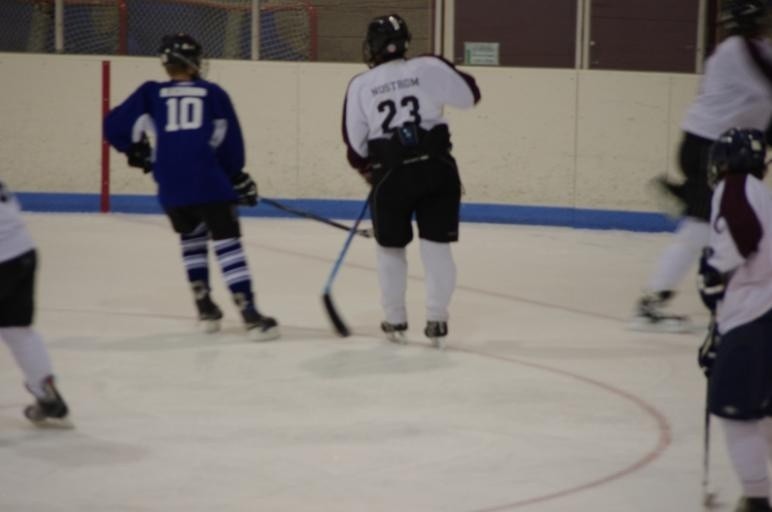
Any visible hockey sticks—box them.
[702,315,720,510]
[322,188,371,336]
[260,194,375,237]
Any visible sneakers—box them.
[380,319,405,333]
[423,321,447,336]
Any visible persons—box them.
[697,127,772,512]
[105,33,278,333]
[629,1,772,325]
[0,184,69,422]
[340,15,481,337]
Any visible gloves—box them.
[234,173,258,204]
[128,133,152,170]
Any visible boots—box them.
[235,292,276,330]
[24,377,69,421]
[189,280,223,321]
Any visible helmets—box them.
[368,14,409,53]
[705,128,770,185]
[157,35,200,72]
